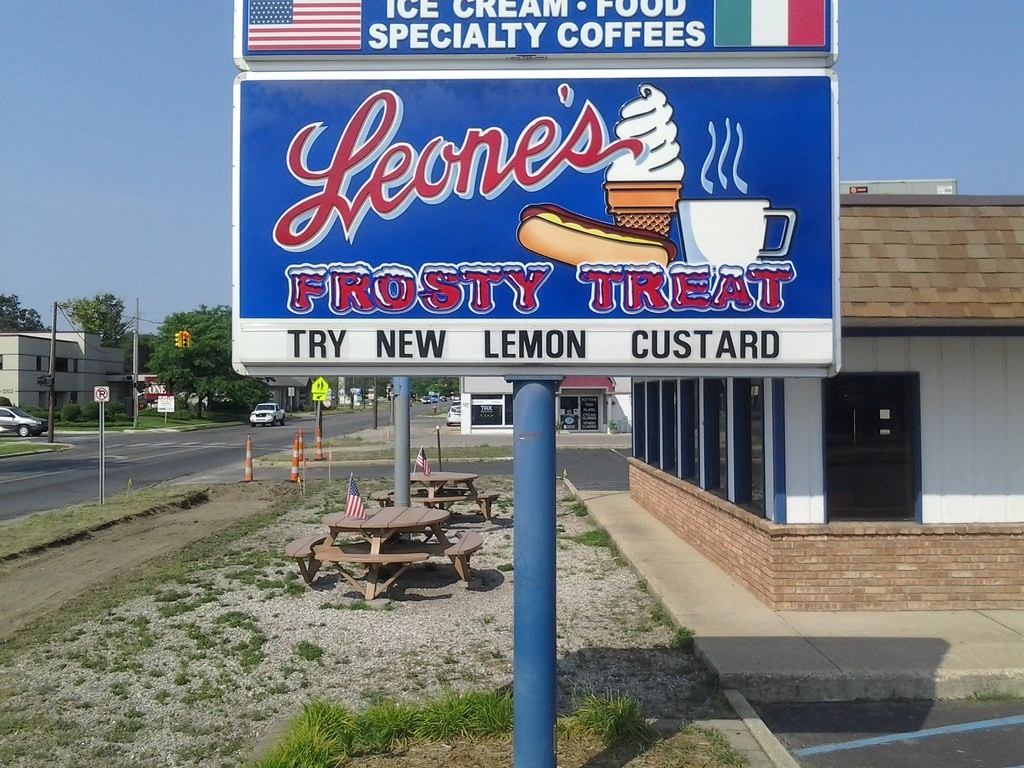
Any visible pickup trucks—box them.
[250,401,286,426]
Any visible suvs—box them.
[448,401,461,413]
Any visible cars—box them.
[178,393,208,411]
[413,395,446,405]
[0,406,48,436]
[446,405,461,428]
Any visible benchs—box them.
[476,491,501,499]
[417,487,470,492]
[314,552,431,562]
[371,489,394,498]
[444,529,483,554]
[285,534,328,557]
[409,495,468,502]
[411,526,449,544]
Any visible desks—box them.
[295,507,469,598]
[382,471,491,519]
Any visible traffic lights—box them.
[174,330,192,348]
[135,383,151,392]
[38,376,53,387]
